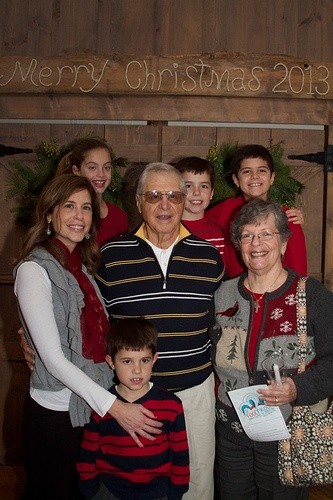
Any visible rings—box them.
[275,396,279,405]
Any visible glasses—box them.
[236,228,280,245]
[139,190,185,205]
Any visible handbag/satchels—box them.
[278,405,333,489]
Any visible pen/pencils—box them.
[273,363,283,386]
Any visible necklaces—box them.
[247,270,282,314]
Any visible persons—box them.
[69,137,129,247]
[75,316,190,500]
[12,173,165,499]
[206,144,309,280]
[210,198,333,499]
[18,162,232,500]
[174,156,306,276]
